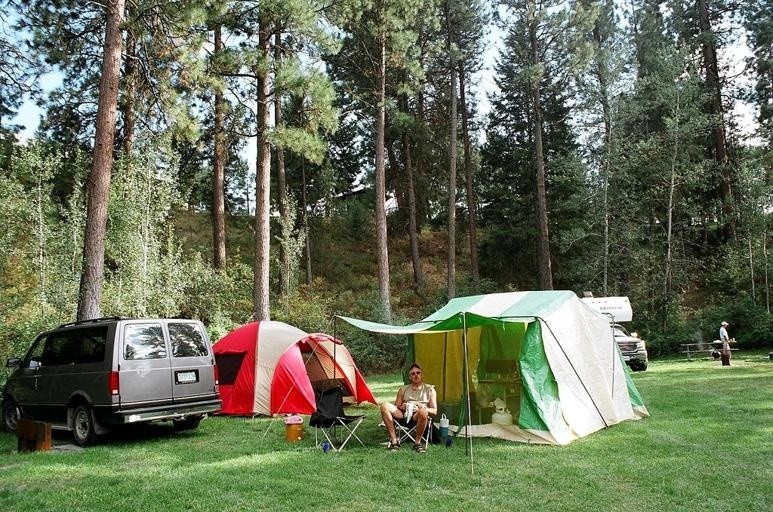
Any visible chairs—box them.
[308,386,367,453]
[386,383,439,452]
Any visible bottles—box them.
[438,412,448,439]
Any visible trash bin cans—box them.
[284,416,304,443]
[17,421,51,453]
[768,352,773,360]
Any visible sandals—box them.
[411,443,426,455]
[386,442,401,451]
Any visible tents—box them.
[334,288,652,447]
[211,320,379,416]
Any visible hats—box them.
[721,321,729,326]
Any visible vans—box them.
[4,316,221,450]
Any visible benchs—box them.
[680,340,740,361]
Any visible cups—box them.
[321,442,328,454]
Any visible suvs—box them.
[577,323,648,372]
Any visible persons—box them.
[380,363,438,453]
[719,321,731,365]
